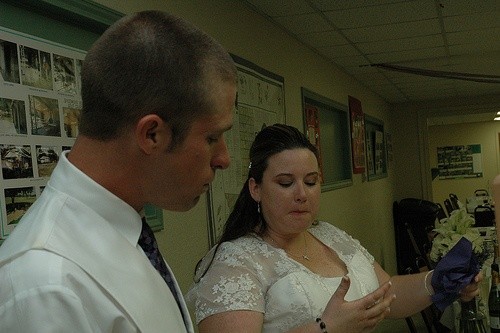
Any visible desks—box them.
[461,196,496,217]
[465,227,497,328]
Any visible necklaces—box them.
[262,231,310,260]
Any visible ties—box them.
[138,215,189,333]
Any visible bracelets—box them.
[316,318,328,333]
[424,269,434,297]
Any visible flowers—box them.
[435,204,489,263]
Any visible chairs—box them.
[390,188,495,333]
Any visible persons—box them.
[0,13,240,333]
[187,122,485,333]
